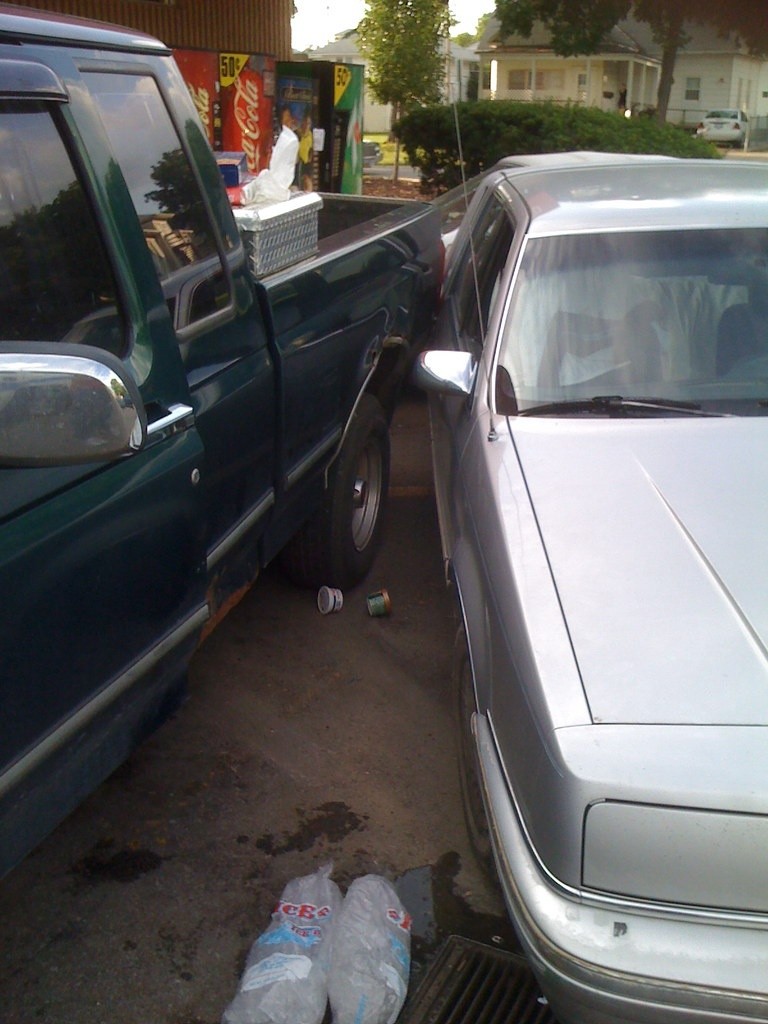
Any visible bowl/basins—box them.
[367,589,391,617]
[317,585,343,615]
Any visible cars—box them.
[696,108,750,148]
[415,149,767,1016]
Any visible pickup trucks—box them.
[0,11,447,887]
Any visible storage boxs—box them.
[229,191,325,280]
[212,152,260,205]
